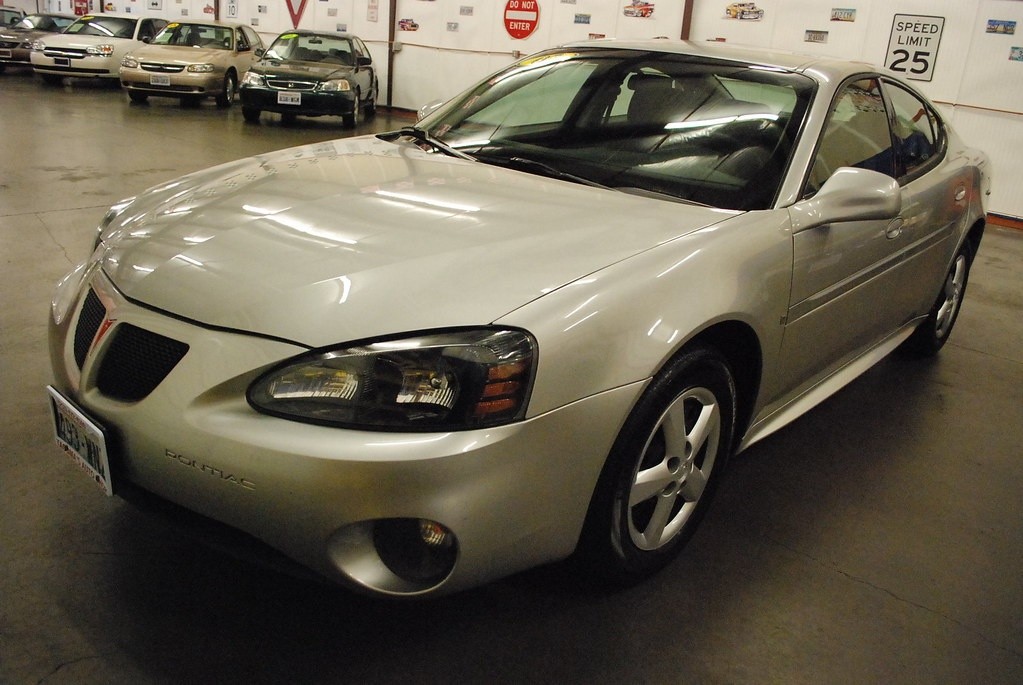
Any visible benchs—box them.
[626,86,774,131]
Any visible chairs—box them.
[292,47,313,60]
[185,32,202,46]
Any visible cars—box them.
[0,14,83,74]
[239,29,379,130]
[119,20,264,108]
[30,12,173,87]
[0,7,27,32]
[623,2,655,18]
[49,37,995,610]
[726,2,764,20]
[398,19,419,31]
